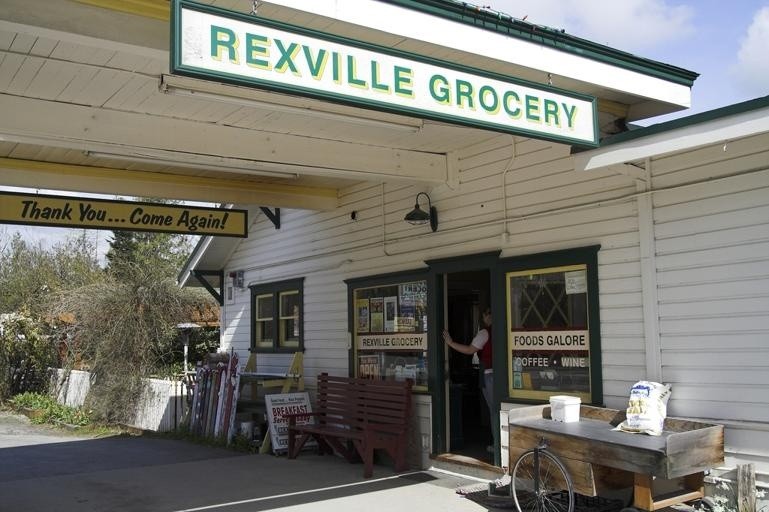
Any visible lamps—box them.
[404,193,438,232]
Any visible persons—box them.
[443,306,495,454]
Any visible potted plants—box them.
[23,394,55,418]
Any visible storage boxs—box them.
[549,396,582,423]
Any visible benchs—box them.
[281,372,413,479]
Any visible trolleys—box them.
[507,399,728,512]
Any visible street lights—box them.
[171,323,202,383]
